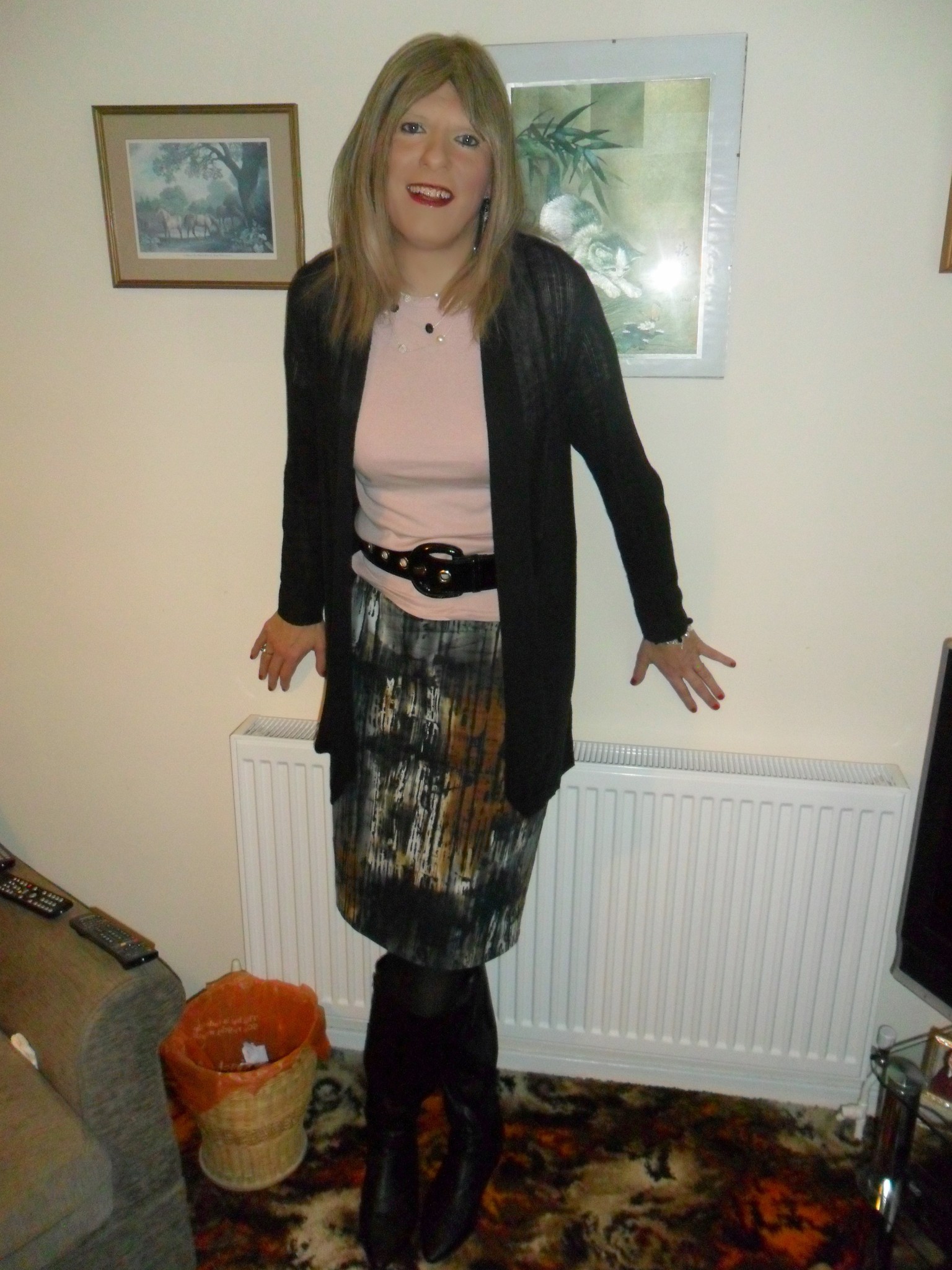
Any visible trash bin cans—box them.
[160,976,327,1191]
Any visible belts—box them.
[361,540,498,599]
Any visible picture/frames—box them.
[89,99,307,292]
[479,26,751,383]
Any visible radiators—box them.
[221,710,919,1131]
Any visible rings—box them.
[695,663,704,672]
[261,647,273,655]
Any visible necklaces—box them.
[382,291,460,352]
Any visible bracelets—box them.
[666,626,693,649]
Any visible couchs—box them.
[0,840,197,1270]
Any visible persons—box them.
[927,1049,952,1102]
[250,33,735,1269]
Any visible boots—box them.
[422,964,504,1262]
[358,952,435,1270]
